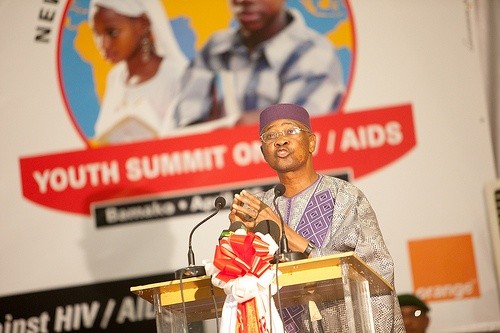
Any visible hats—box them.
[260,103,310,132]
[398,293,430,310]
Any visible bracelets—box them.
[303,240,316,256]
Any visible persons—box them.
[398,294,430,333]
[228,103,405,333]
[88,1,345,137]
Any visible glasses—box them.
[260,127,308,143]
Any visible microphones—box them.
[272,184,307,263]
[175,196,226,281]
